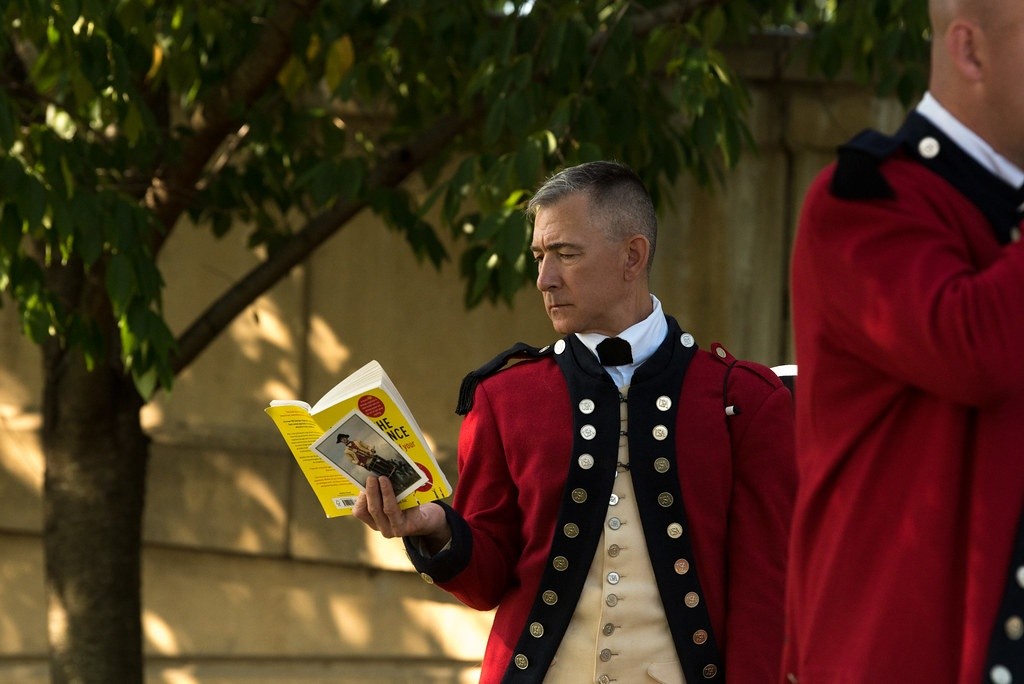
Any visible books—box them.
[264,360,453,518]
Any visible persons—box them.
[355,160,793,684]
[336,434,397,482]
[780,0,1024,684]
